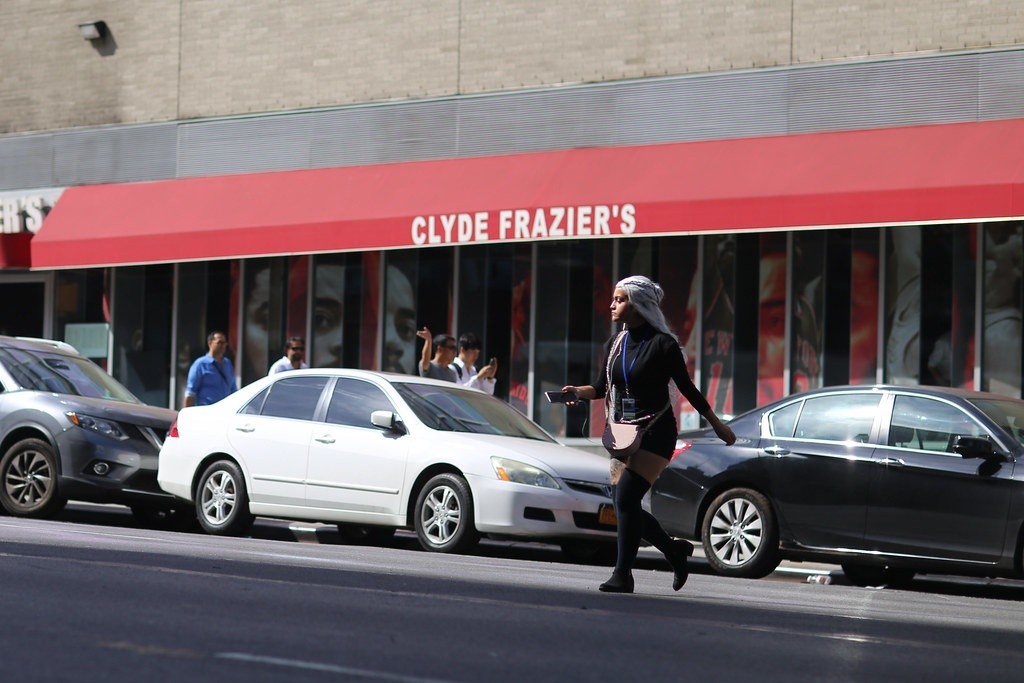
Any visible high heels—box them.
[598,537,696,593]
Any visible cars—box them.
[649,384,1024,587]
[0,332,194,529]
[156,367,657,568]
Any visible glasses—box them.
[440,345,458,351]
[212,341,228,346]
[288,346,304,351]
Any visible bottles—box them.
[806,574,832,585]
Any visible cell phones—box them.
[488,358,497,379]
[544,390,579,403]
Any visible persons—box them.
[417,326,458,384]
[267,337,310,379]
[181,332,237,409]
[384,264,418,378]
[563,275,737,592]
[312,263,344,369]
[246,266,270,381]
[447,334,497,396]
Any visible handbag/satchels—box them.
[602,422,645,456]
